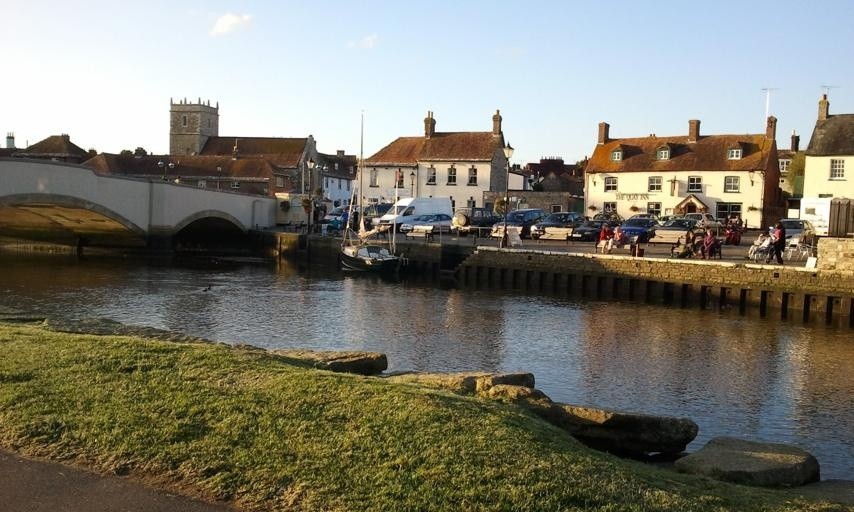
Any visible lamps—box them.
[749,169,755,185]
[591,168,597,186]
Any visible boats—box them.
[341,243,408,271]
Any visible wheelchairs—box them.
[746,242,778,264]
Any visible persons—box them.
[684,230,697,258]
[745,231,772,261]
[339,207,348,233]
[765,221,785,264]
[700,228,716,260]
[769,226,776,241]
[351,206,359,231]
[734,215,743,230]
[607,226,624,255]
[600,222,615,254]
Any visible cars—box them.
[776,219,816,247]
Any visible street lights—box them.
[306,158,315,232]
[410,172,416,196]
[502,143,514,243]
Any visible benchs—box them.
[492,225,522,239]
[672,235,722,259]
[282,219,303,231]
[405,224,434,242]
[648,230,694,245]
[539,226,574,244]
[595,234,638,254]
